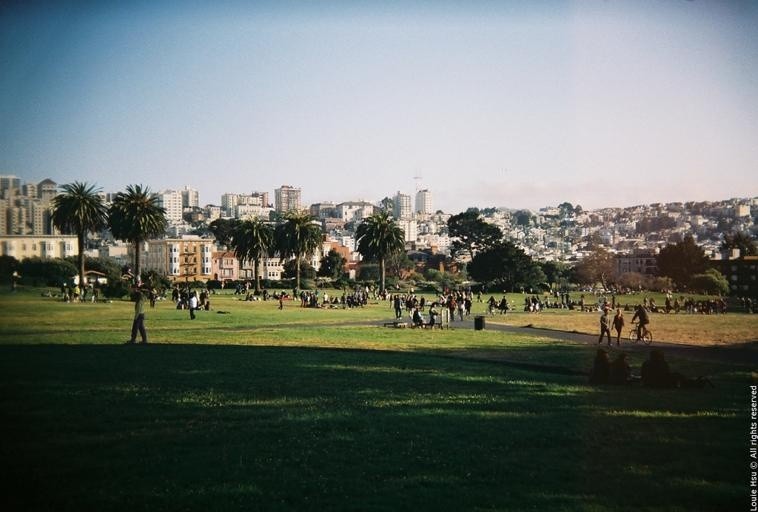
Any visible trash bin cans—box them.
[474,315,486,330]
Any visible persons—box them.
[611,309,626,345]
[62,274,210,343]
[519,285,612,315]
[631,303,650,341]
[598,306,613,347]
[734,296,754,312]
[234,282,509,329]
[642,289,727,314]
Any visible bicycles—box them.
[629,320,653,345]
